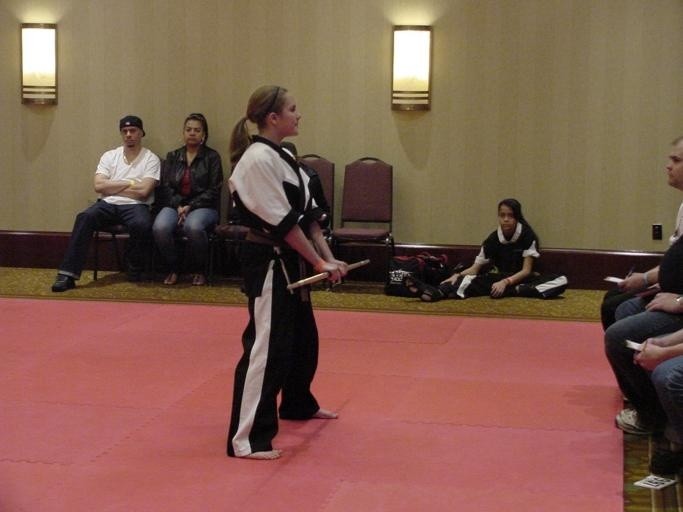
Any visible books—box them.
[604,272,628,291]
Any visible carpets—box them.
[0,298,624,511]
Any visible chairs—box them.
[93,184,161,279]
[299,152,334,291]
[330,156,396,291]
[219,163,250,282]
[162,160,223,286]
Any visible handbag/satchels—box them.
[384,251,453,295]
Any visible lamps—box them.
[391,24,433,112]
[20,22,58,106]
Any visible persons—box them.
[632,325,682,473]
[438,199,569,301]
[597,135,682,333]
[278,140,332,230]
[151,112,222,287]
[227,85,347,461]
[51,115,160,295]
[600,234,683,435]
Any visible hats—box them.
[120,116,146,136]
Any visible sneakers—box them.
[402,275,439,302]
[164,272,177,285]
[192,273,205,285]
[53,273,76,292]
[613,409,651,435]
[490,285,516,299]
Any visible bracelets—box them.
[642,270,648,286]
[133,176,141,183]
[458,271,463,278]
[504,278,512,286]
[122,178,134,190]
[675,295,683,307]
